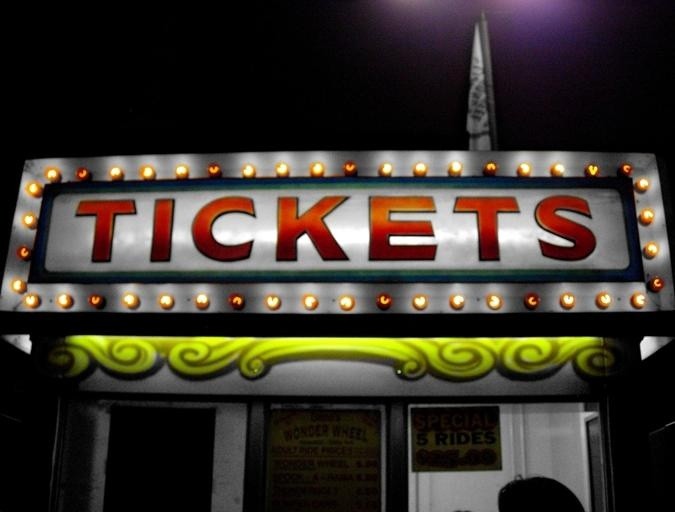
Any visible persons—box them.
[448,471,586,512]
